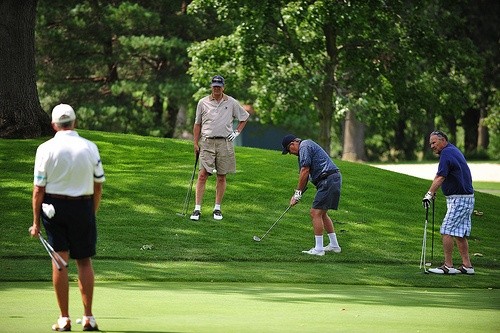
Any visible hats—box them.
[281,135,296,155]
[52,104,76,123]
[212,76,225,86]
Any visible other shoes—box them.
[190,210,201,220]
[213,210,223,220]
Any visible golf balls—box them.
[253,235,257,240]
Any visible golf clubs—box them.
[175,147,201,217]
[419,192,436,274]
[28,227,69,272]
[252,186,308,242]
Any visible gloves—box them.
[227,130,240,141]
[42,203,55,219]
[422,191,432,209]
[290,190,302,206]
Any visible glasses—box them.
[287,144,291,153]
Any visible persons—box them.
[29,103,105,331]
[280,134,341,256]
[190,75,250,220]
[422,130,476,275]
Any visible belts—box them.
[44,195,93,199]
[205,137,226,140]
[314,170,336,185]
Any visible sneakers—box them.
[323,244,341,253]
[448,265,474,274]
[82,320,100,330]
[52,320,71,331]
[302,248,325,256]
[428,264,454,274]
